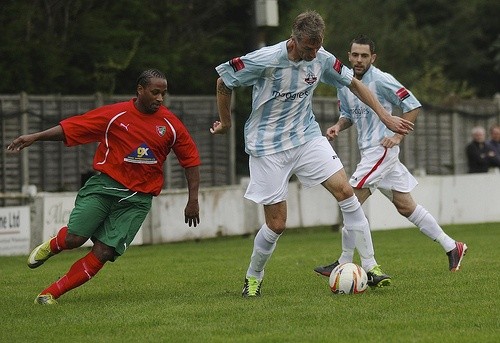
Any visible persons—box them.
[209,9,393,299]
[463,128,500,175]
[7,68,201,307]
[487,125,500,164]
[314,33,468,277]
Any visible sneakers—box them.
[242,274,263,298]
[366,265,391,288]
[446,241,468,272]
[34,293,58,306]
[27,235,59,269]
[314,260,340,277]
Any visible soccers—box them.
[329,262,368,295]
